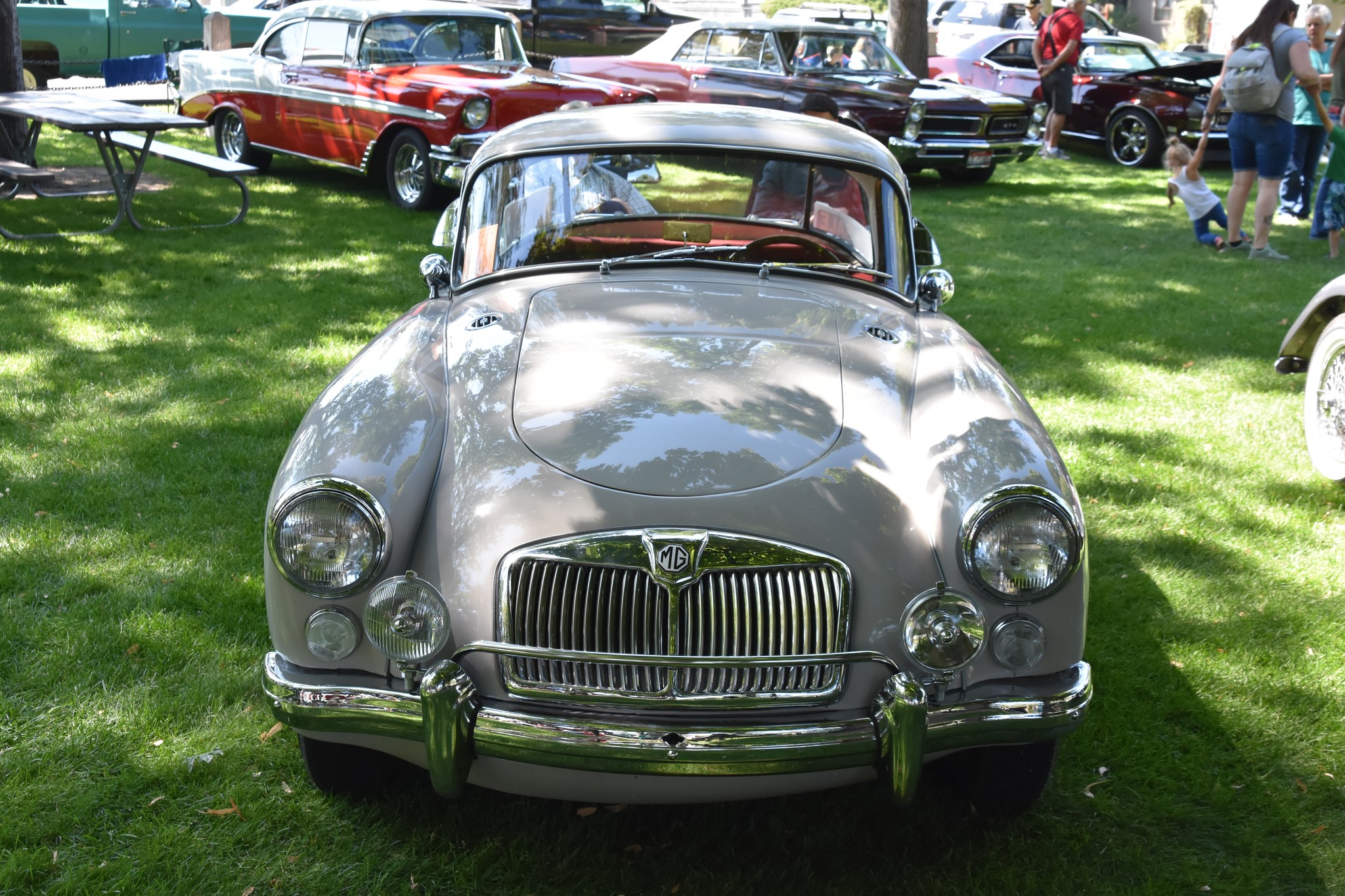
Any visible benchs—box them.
[0,157,56,238]
[546,236,872,281]
[87,130,259,233]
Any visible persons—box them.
[792,33,880,70]
[1164,135,1253,250]
[518,101,659,241]
[1031,0,1088,160]
[751,93,866,246]
[1013,0,1048,56]
[1277,3,1345,262]
[1201,0,1321,260]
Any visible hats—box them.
[1024,0,1040,8]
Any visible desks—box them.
[0,89,209,232]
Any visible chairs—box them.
[360,46,416,66]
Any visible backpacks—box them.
[1221,27,1295,113]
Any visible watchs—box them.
[1203,109,1213,119]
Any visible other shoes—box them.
[1215,237,1225,250]
[1244,236,1251,243]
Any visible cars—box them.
[259,100,1096,835]
[546,16,1045,185]
[1273,272,1345,480]
[170,0,662,215]
[409,1,1228,171]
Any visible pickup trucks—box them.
[18,0,297,91]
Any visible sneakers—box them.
[1038,141,1064,155]
[1224,240,1252,252]
[1042,150,1070,162]
[1248,244,1290,261]
